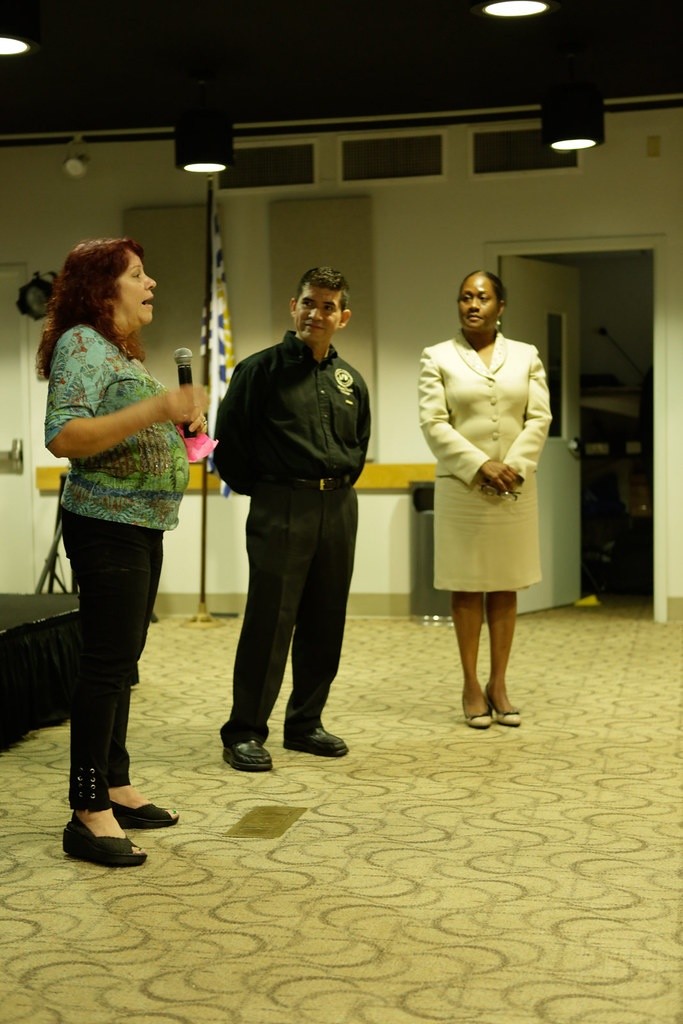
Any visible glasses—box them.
[481,483,522,502]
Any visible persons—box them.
[210,267,373,773]
[417,269,552,729]
[34,237,207,867]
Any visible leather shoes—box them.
[283,725,349,757]
[223,739,274,771]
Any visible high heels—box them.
[484,684,520,727]
[462,691,491,729]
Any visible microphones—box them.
[173,347,198,439]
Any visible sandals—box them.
[110,800,180,830]
[62,810,147,866]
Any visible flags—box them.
[200,171,238,499]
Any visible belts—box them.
[257,474,350,491]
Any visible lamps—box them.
[0,0,33,56]
[481,0,550,19]
[539,42,605,151]
[174,85,234,174]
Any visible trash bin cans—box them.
[407,480,455,616]
